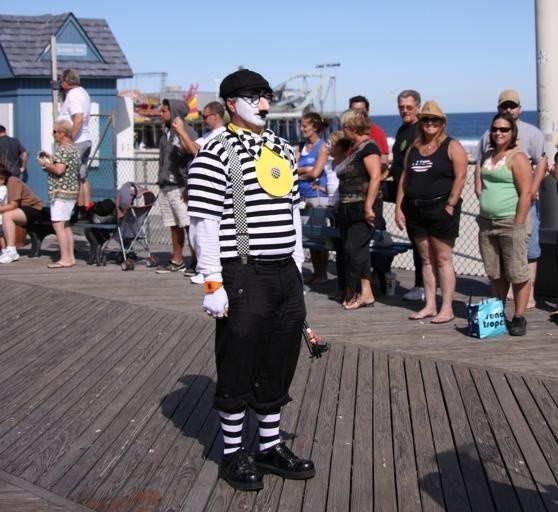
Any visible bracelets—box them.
[200,281,225,293]
[446,201,456,210]
[41,161,48,171]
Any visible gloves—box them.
[201,272,230,320]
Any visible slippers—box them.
[328,289,375,311]
[430,315,456,325]
[47,260,78,269]
[408,311,439,321]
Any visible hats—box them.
[415,99,448,124]
[498,88,520,106]
[218,68,272,99]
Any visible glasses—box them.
[490,126,513,133]
[235,92,273,106]
[399,105,414,111]
[201,112,215,121]
[419,116,443,123]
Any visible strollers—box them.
[92,179,164,270]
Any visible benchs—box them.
[297,207,416,298]
[20,187,125,269]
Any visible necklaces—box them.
[309,141,315,151]
[424,142,439,151]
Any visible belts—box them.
[404,192,450,208]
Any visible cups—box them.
[384,272,396,294]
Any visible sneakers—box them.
[402,285,443,304]
[190,273,205,285]
[153,258,187,275]
[182,259,199,277]
[0,248,20,264]
[301,273,329,285]
[509,314,528,337]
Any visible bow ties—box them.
[231,127,292,162]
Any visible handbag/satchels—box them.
[465,288,510,340]
[346,216,377,251]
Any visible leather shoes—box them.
[252,440,318,481]
[217,445,265,492]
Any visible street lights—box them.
[314,63,341,114]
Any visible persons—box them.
[309,129,352,302]
[178,64,313,490]
[332,106,383,309]
[1,167,10,253]
[548,149,557,326]
[388,90,425,302]
[155,98,199,278]
[473,113,531,338]
[34,117,82,269]
[473,86,548,310]
[349,94,390,233]
[292,112,335,285]
[0,165,47,265]
[52,67,93,208]
[393,99,468,324]
[170,101,226,286]
[0,124,29,185]
